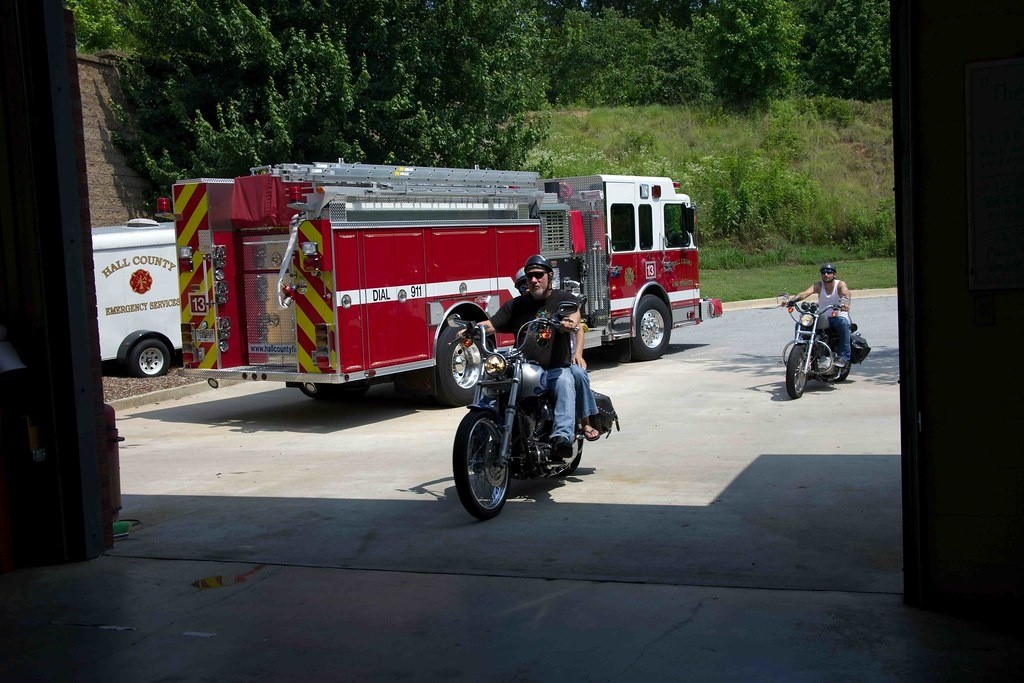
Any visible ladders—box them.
[250,159,542,209]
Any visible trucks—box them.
[90,218,183,379]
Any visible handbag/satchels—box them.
[851,334,871,364]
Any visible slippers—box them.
[582,427,600,442]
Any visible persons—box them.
[782,263,852,368]
[456,254,600,458]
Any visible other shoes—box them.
[554,437,572,458]
[834,357,848,367]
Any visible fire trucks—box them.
[153,156,725,409]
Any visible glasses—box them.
[519,285,529,292]
[821,269,834,274]
[526,271,548,279]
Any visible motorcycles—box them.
[446,302,594,520]
[779,291,859,400]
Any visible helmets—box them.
[515,267,526,288]
[820,264,836,273]
[524,254,553,273]
